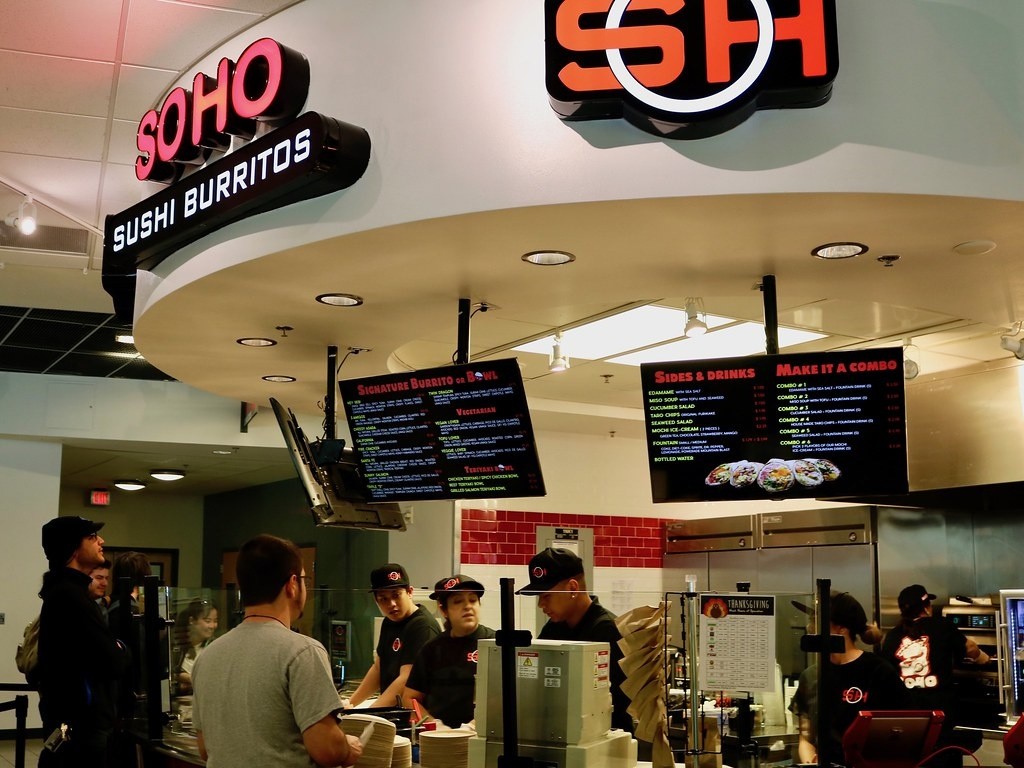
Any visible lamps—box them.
[5,191,38,235]
[549,330,570,373]
[685,297,707,338]
[1000,321,1024,360]
[900,337,921,380]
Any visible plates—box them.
[705,459,841,493]
[337,714,477,768]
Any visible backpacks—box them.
[15,616,40,674]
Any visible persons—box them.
[192,535,364,768]
[398,574,497,732]
[514,547,650,760]
[34,516,218,768]
[786,584,992,768]
[341,562,443,706]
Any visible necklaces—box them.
[241,614,286,627]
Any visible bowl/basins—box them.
[337,679,381,700]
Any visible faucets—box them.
[333,660,345,684]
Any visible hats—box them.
[791,589,868,633]
[42,516,106,568]
[368,564,409,593]
[516,547,582,596]
[898,584,937,610]
[440,574,485,597]
[429,578,447,600]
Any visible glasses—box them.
[88,532,97,540]
[296,573,311,583]
